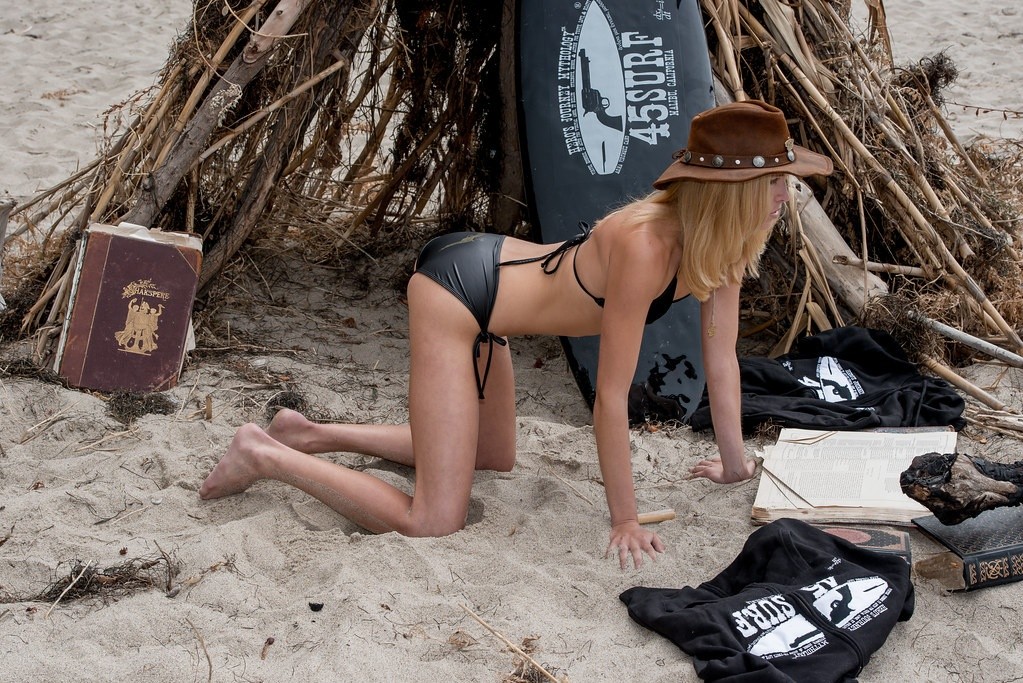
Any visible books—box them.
[51,221,203,394]
[752,427,957,527]
[912,505,1023,592]
[807,523,911,578]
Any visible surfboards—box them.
[511,0,723,434]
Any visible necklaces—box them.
[697,282,716,338]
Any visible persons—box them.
[198,101,833,569]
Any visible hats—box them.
[653,100,833,190]
